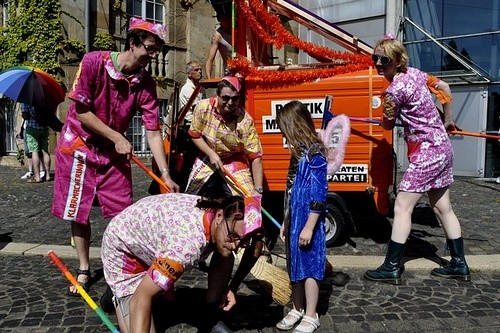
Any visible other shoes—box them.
[46,177,53,182]
[27,178,42,183]
[40,170,46,179]
[21,172,33,179]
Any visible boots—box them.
[365,237,406,285]
[433,236,471,280]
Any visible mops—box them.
[320,94,499,139]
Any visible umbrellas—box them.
[0,66,65,126]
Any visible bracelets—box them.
[160,168,170,173]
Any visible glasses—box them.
[219,95,240,102]
[372,54,393,66]
[225,220,249,248]
[139,40,163,55]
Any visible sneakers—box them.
[293,313,319,333]
[277,309,304,330]
[212,321,235,333]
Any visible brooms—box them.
[131,154,293,306]
[222,169,333,275]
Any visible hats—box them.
[222,76,242,92]
[244,196,262,236]
[128,18,167,43]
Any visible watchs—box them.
[254,187,264,193]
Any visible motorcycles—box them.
[150,64,398,250]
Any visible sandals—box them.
[67,269,93,296]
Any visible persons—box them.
[21,103,51,183]
[51,17,178,296]
[178,61,205,130]
[101,193,262,333]
[364,33,471,285]
[206,8,232,79]
[276,100,329,333]
[185,76,264,196]
